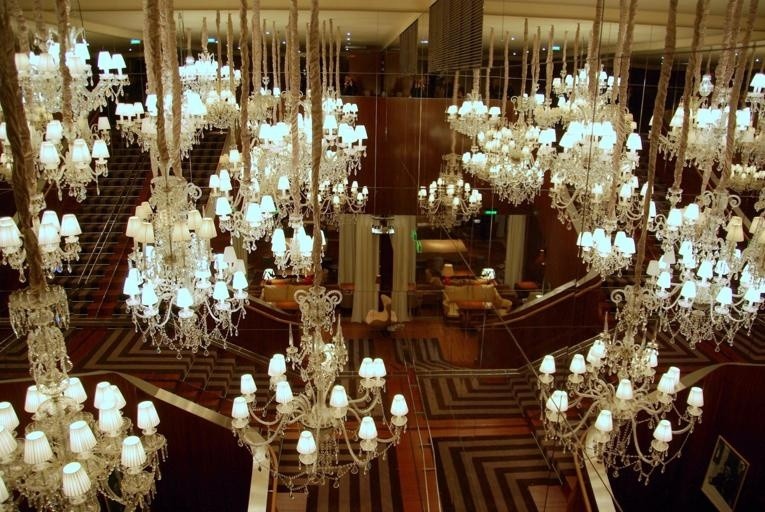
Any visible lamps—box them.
[322,29,369,226]
[0,4,67,204]
[446,69,499,140]
[462,30,499,178]
[70,4,328,166]
[1,4,82,282]
[576,2,635,279]
[0,2,170,512]
[491,4,765,231]
[28,2,63,117]
[537,2,703,486]
[229,0,409,501]
[417,72,482,231]
[122,1,248,358]
[49,2,111,201]
[324,18,369,175]
[266,2,327,278]
[639,1,765,353]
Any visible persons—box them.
[709,463,737,508]
[341,73,514,100]
